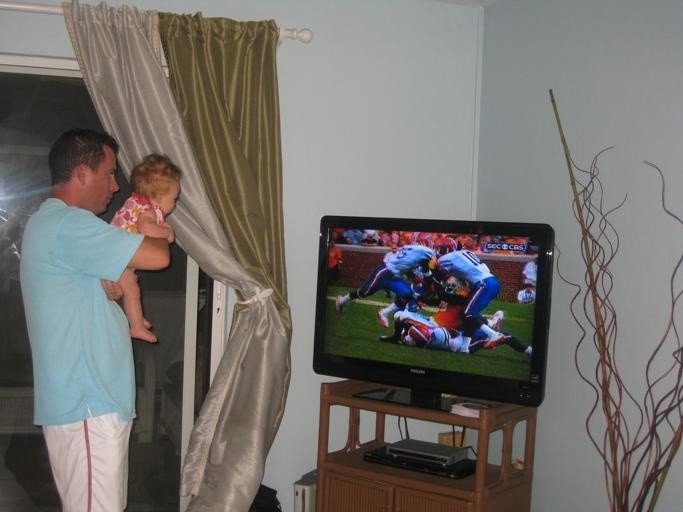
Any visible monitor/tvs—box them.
[313,216,554,418]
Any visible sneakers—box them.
[484,333,504,349]
[491,310,503,331]
[380,335,401,343]
[377,309,389,328]
[336,295,343,313]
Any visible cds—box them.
[462,400,491,410]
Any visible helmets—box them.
[408,324,430,343]
[416,232,473,253]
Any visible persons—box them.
[328,227,538,356]
[18,128,171,511]
[105,152,183,343]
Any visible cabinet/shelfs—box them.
[317,383,537,512]
